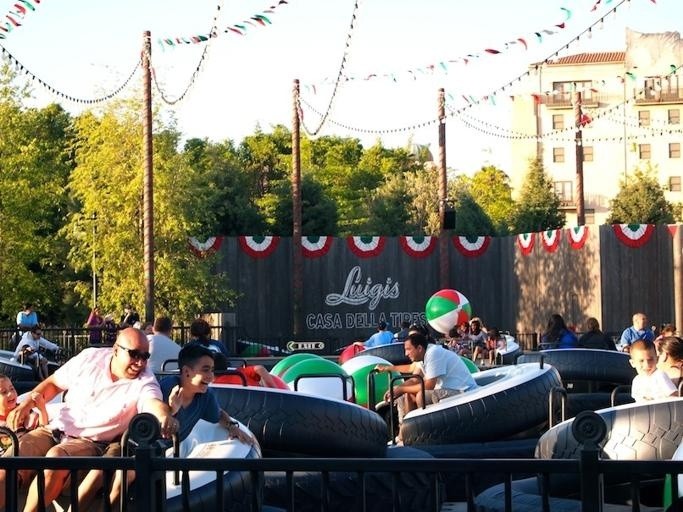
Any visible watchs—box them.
[228,420,240,430]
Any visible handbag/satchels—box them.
[9,313,23,343]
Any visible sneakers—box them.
[387,437,401,445]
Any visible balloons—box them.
[425,289,471,335]
[268,353,322,379]
[279,358,357,403]
[239,346,271,357]
[662,436,683,511]
[340,355,405,409]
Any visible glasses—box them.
[115,343,151,359]
[35,332,42,335]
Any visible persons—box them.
[620,313,654,353]
[66,343,254,512]
[105,305,139,340]
[580,318,618,352]
[376,327,427,375]
[1,375,49,458]
[654,324,677,355]
[383,334,477,445]
[8,303,39,346]
[140,322,154,334]
[146,317,182,370]
[85,308,104,347]
[658,337,682,396]
[541,315,579,348]
[470,316,517,366]
[353,321,394,349]
[628,340,679,403]
[14,326,60,381]
[213,352,278,388]
[180,319,227,358]
[1,327,179,512]
